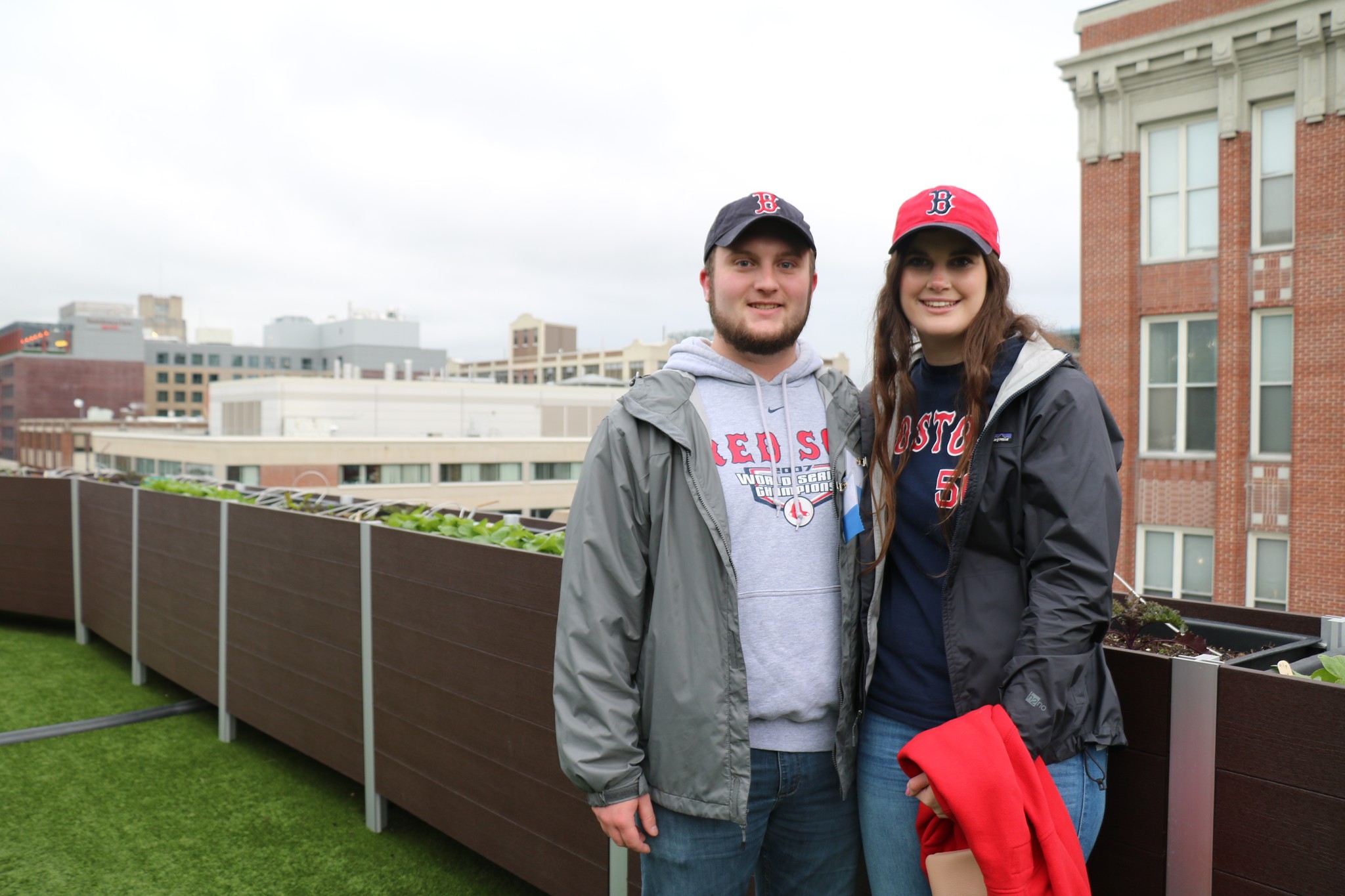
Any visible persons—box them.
[845,185,1129,895]
[553,192,864,895]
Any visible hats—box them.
[704,192,816,259]
[888,185,1000,257]
